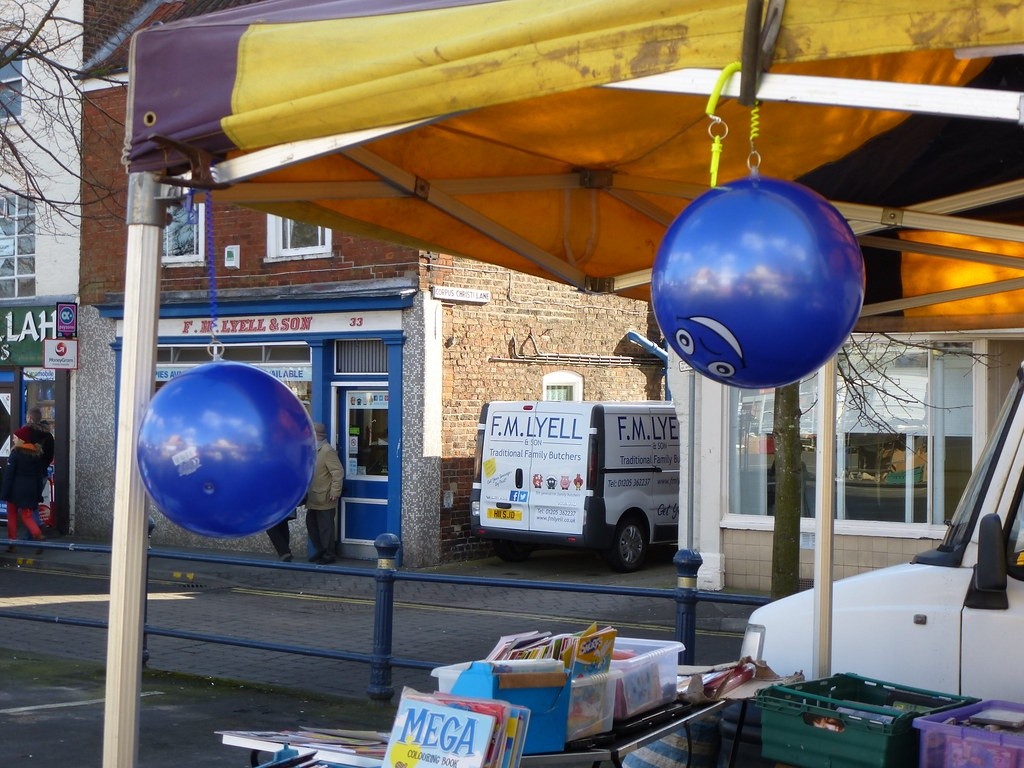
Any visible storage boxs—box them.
[755,672,982,768]
[431,637,686,755]
[912,700,1024,768]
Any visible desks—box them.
[222,700,749,768]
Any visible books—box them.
[382,621,616,768]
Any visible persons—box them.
[305,423,344,563]
[0,406,57,543]
[267,508,298,561]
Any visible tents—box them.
[101,0,1024,768]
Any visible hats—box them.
[314,423,327,436]
[14,425,30,442]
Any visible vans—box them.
[468,399,678,570]
[737,360,1024,708]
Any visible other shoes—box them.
[5,547,17,552]
[36,535,46,554]
[278,554,294,562]
[308,554,336,564]
